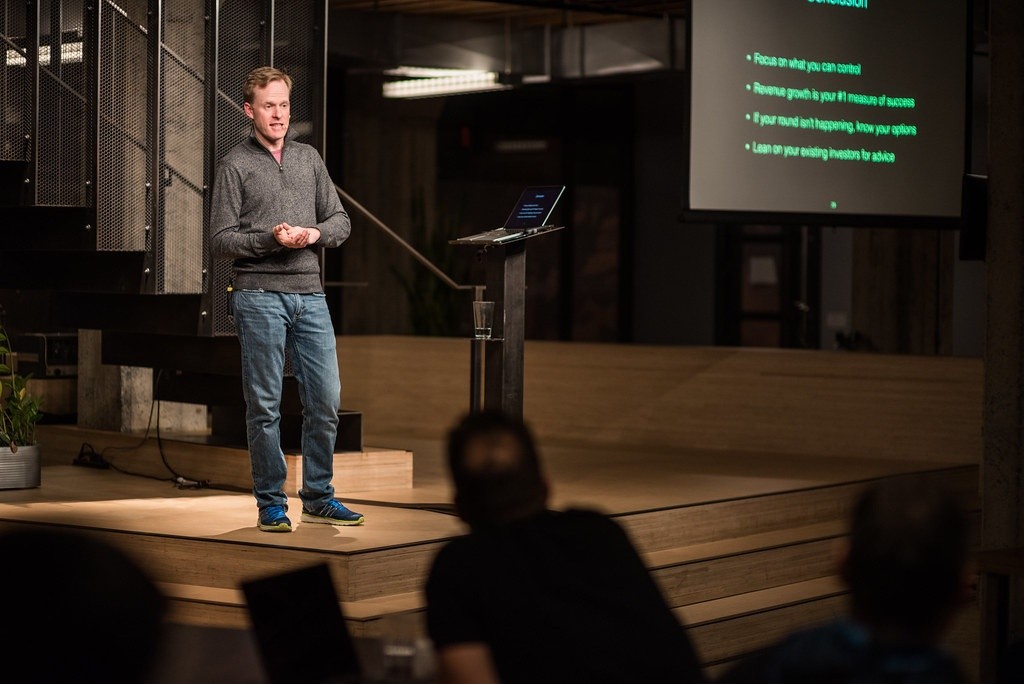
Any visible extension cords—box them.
[73,459,110,469]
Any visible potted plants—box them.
[0,326,46,490]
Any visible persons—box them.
[424,409,707,684]
[716,488,977,684]
[0,524,168,684]
[210,66,365,530]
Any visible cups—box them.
[473,301,495,338]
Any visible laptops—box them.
[457,184,566,243]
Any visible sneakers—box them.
[257,505,293,532]
[299,498,366,526]
